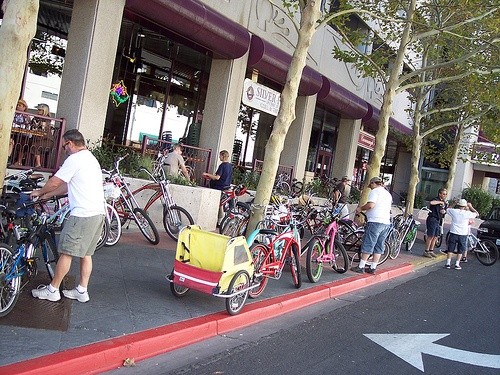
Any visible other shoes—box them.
[460,257,467,262]
[430,252,438,257]
[352,266,366,273]
[365,268,377,274]
[446,265,451,269]
[439,249,448,255]
[425,252,432,258]
[455,266,462,269]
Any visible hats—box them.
[368,176,382,188]
[457,199,467,206]
[342,176,351,181]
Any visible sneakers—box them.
[32,285,61,302]
[62,288,90,303]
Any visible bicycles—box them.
[219,172,433,301]
[424,214,443,247]
[446,220,499,266]
[1,150,196,317]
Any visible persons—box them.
[202,150,233,228]
[444,198,479,270]
[162,143,192,186]
[29,128,105,302]
[333,176,351,219]
[8,99,54,167]
[440,199,475,263]
[350,177,393,274]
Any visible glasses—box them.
[62,142,70,150]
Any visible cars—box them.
[477,205,500,255]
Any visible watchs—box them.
[423,188,447,258]
